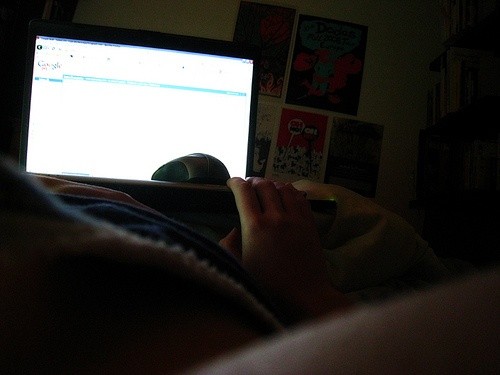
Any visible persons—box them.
[0,170,499,375]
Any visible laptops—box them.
[17,18,338,213]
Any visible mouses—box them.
[152,154,231,186]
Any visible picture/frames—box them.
[270,103,331,183]
[248,96,283,177]
[323,115,385,200]
[283,13,368,116]
[233,1,297,102]
